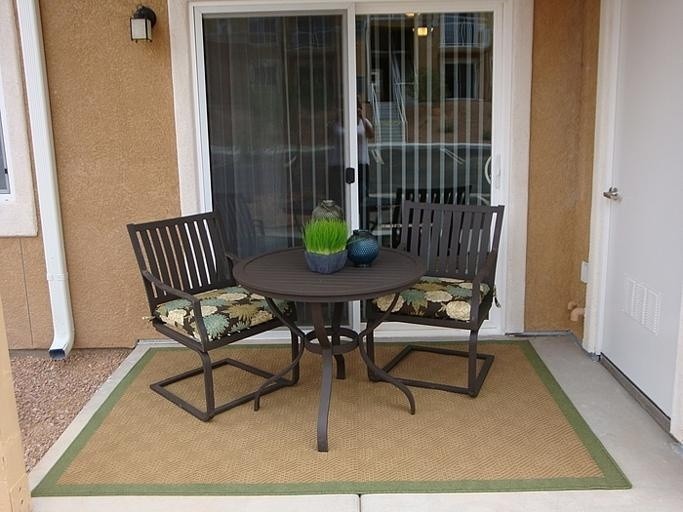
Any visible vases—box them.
[311,200,344,222]
[346,229,379,267]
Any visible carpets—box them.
[27,337,633,499]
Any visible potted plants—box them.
[299,217,350,275]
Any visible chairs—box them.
[209,192,273,261]
[284,163,375,237]
[380,184,473,260]
[127,210,301,425]
[363,196,507,399]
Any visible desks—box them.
[230,242,427,454]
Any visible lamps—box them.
[410,23,434,38]
[127,3,156,44]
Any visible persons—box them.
[331,95,375,235]
